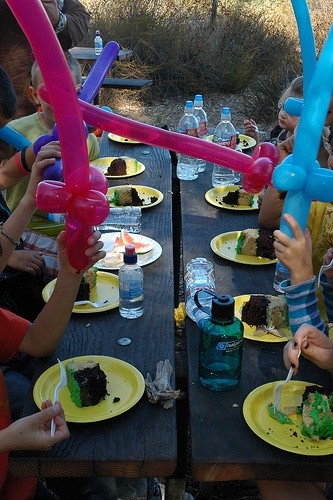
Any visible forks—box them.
[50,358,67,438]
[245,120,266,134]
[74,299,111,308]
[318,258,333,289]
[273,336,308,414]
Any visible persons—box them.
[245,77,333,500]
[0,1,194,500]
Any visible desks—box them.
[7,130,333,499]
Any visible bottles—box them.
[176,101,198,180]
[211,106,235,187]
[273,258,290,293]
[47,208,142,233]
[94,30,103,56]
[184,257,216,332]
[233,132,242,183]
[118,244,144,320]
[192,95,208,173]
[194,288,244,391]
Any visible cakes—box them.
[107,158,137,175]
[236,229,278,260]
[111,187,158,206]
[241,295,289,329]
[64,359,108,409]
[223,187,258,207]
[300,385,333,440]
[236,133,248,147]
[75,267,99,302]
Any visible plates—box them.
[103,185,164,209]
[203,134,256,150]
[88,156,146,179]
[210,231,279,265]
[33,355,146,424]
[205,185,266,210]
[242,381,333,456]
[91,231,162,269]
[42,270,129,313]
[232,294,293,342]
[108,128,160,144]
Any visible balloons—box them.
[272,0,333,238]
[37,83,278,193]
